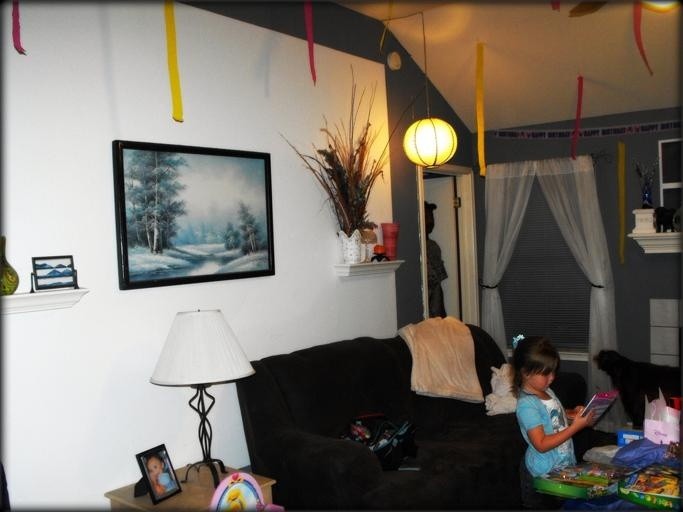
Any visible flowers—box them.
[272,60,428,236]
[631,150,664,194]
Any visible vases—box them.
[641,191,653,209]
[0,234,19,295]
[339,222,400,266]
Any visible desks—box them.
[104,460,274,512]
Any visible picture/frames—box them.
[29,254,80,292]
[110,136,277,293]
[134,444,182,505]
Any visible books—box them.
[582,390,618,425]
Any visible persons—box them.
[147,453,176,497]
[512,336,596,510]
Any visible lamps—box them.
[148,305,255,488]
[401,8,461,168]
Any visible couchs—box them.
[234,321,590,510]
